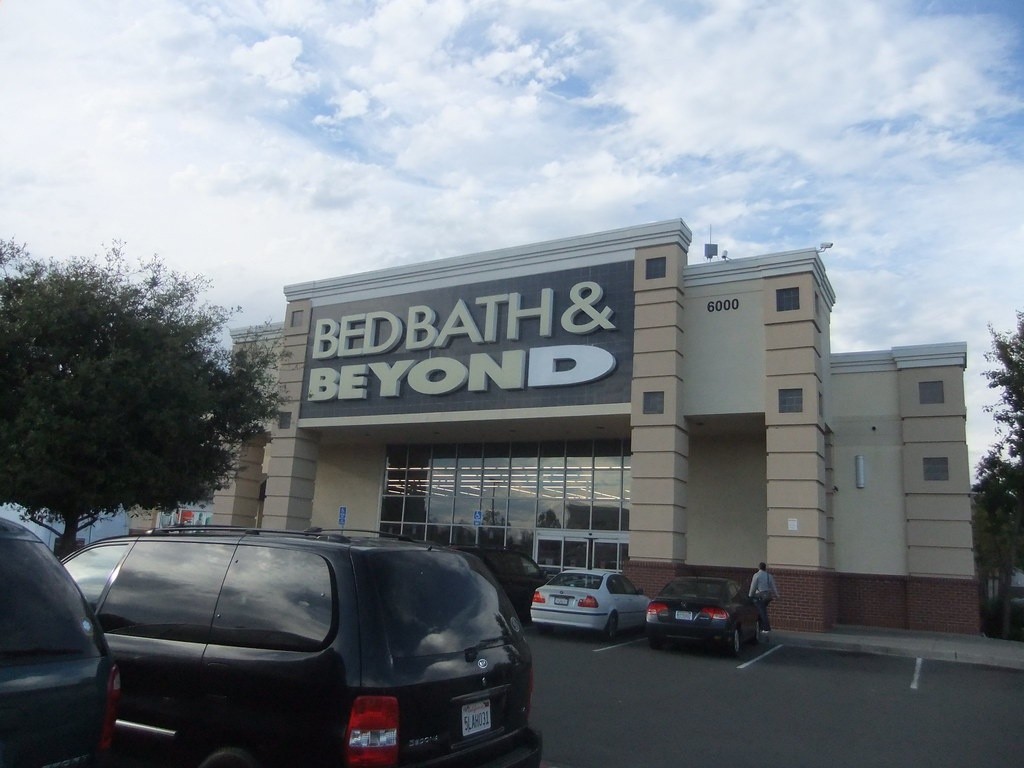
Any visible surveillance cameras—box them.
[721,250,728,259]
[820,242,833,248]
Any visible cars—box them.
[529,569,651,640]
[645,577,761,654]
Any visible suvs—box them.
[448,544,549,611]
[0,517,122,768]
[59,526,543,768]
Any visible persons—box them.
[748,562,780,633]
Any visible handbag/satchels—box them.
[757,590,775,603]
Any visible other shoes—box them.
[759,629,769,634]
